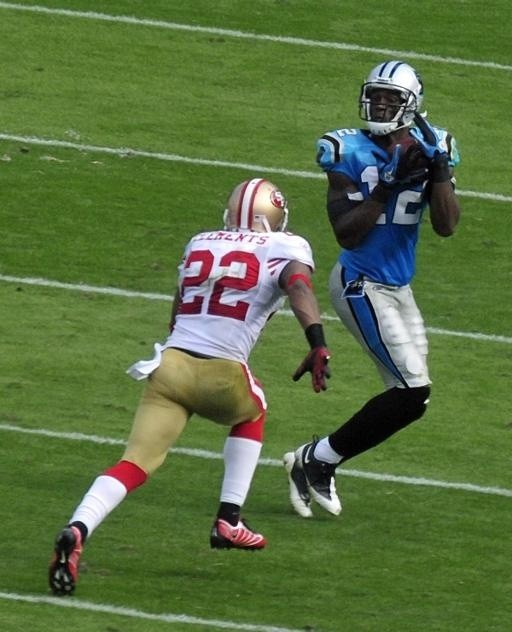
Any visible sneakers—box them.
[207,517,268,551]
[45,524,83,597]
[283,440,344,517]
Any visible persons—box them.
[280,59,463,521]
[47,175,333,597]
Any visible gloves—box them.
[292,346,334,393]
[371,109,451,192]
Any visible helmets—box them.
[357,60,428,137]
[223,176,289,232]
